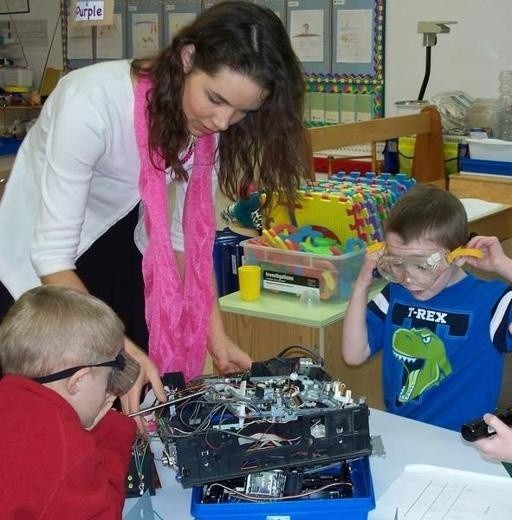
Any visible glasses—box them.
[366,241,484,286]
[31,348,140,396]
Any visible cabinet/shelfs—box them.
[448,173,511,210]
[213,197,512,413]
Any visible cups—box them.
[238,265,261,301]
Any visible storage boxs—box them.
[237,236,386,305]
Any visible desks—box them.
[122,401,512,520]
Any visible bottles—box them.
[298,289,320,309]
[489,70,512,142]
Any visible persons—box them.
[471,413,512,464]
[1,1,311,438]
[342,181,512,432]
[0,285,138,520]
